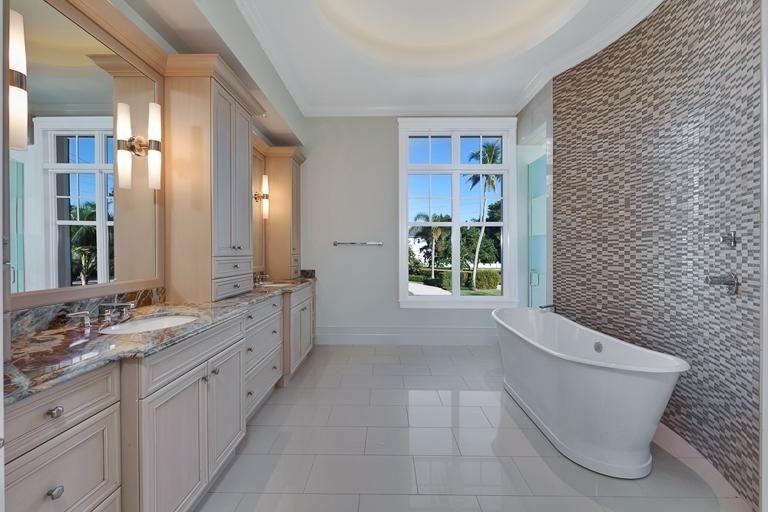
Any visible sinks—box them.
[99,312,200,335]
[258,281,295,287]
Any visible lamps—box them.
[256,174,269,220]
[10,9,28,152]
[115,101,136,190]
[133,104,162,191]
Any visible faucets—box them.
[256,275,270,279]
[99,302,136,315]
[539,304,557,312]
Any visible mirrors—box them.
[252,149,266,267]
[10,0,166,295]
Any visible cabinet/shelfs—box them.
[211,77,253,302]
[290,286,314,373]
[138,315,246,512]
[3,361,122,512]
[242,295,284,424]
[267,159,302,279]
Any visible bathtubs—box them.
[491,308,690,481]
